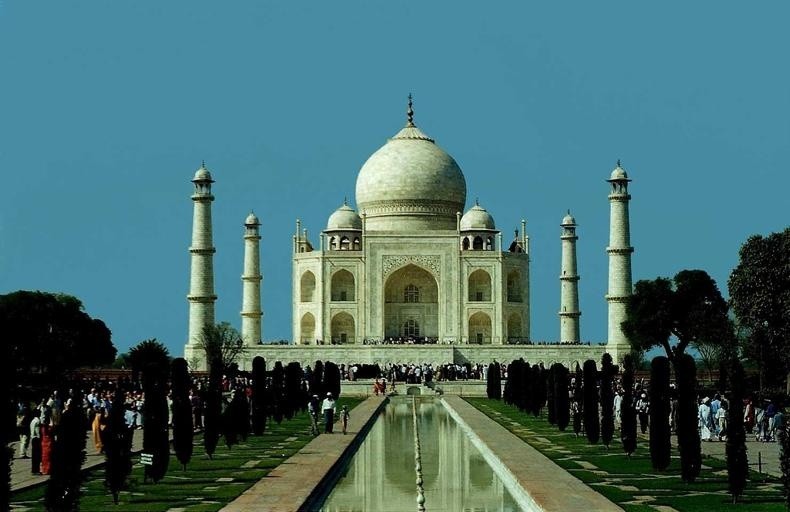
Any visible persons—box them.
[380,379,387,395]
[321,391,336,434]
[339,404,351,436]
[337,360,509,384]
[568,369,789,446]
[307,394,321,437]
[13,374,273,477]
[254,335,610,346]
[373,379,379,395]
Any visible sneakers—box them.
[311,428,348,435]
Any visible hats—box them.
[312,392,332,398]
